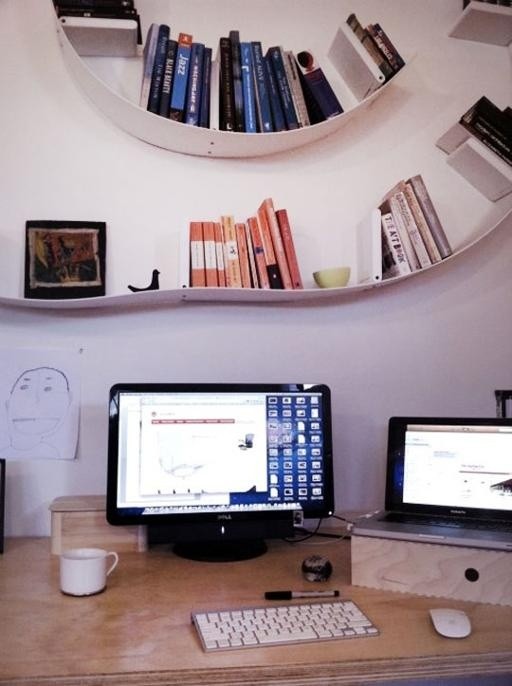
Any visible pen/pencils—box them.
[265,590,339,599]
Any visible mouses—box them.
[428,605,473,639]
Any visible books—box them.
[188,197,305,293]
[458,93,510,180]
[346,12,403,81]
[379,173,453,280]
[140,23,344,133]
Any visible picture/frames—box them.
[25,219,107,298]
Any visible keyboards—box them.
[189,597,381,653]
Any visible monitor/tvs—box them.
[104,381,335,562]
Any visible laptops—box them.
[345,414,512,551]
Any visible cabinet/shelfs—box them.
[0,0,511,310]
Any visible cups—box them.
[59,548,119,597]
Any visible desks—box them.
[0,525,511,685]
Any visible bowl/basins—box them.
[313,267,351,289]
